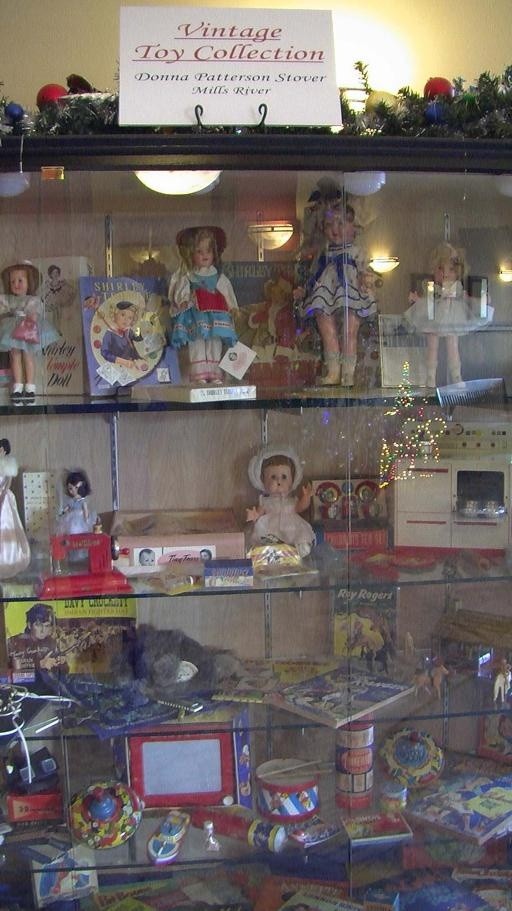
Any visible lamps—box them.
[369,256,399,273]
[247,222,294,251]
[343,171,385,198]
[133,170,221,197]
[0,171,29,199]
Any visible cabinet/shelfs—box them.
[0,135,510,910]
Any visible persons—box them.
[56,470,101,561]
[139,549,156,566]
[200,548,212,563]
[245,440,317,557]
[173,225,240,385]
[403,243,495,389]
[1,437,32,580]
[1,260,60,407]
[294,200,376,387]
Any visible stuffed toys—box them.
[144,631,242,701]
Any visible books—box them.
[345,770,512,847]
[212,585,414,728]
